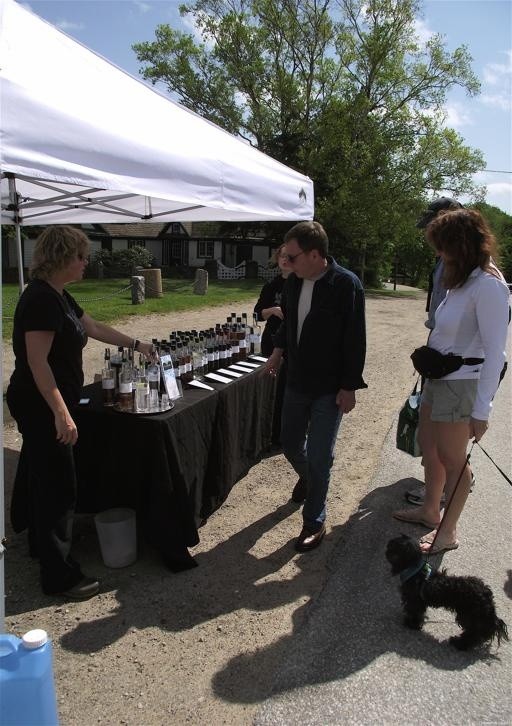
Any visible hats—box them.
[414,197,464,229]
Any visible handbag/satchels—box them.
[409,346,462,380]
[396,398,423,457]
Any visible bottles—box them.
[409,395,420,410]
[100,310,263,412]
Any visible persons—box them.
[263,218,369,553]
[384,208,512,558]
[252,243,292,453]
[1,222,162,601]
[402,194,511,507]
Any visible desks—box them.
[61,353,284,556]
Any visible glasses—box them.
[286,250,306,265]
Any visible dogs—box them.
[384,533,509,651]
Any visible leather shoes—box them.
[295,522,326,551]
[42,570,101,599]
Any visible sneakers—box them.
[404,484,447,505]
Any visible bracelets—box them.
[132,334,140,352]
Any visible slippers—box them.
[419,535,459,554]
[392,510,439,530]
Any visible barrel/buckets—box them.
[95,510,137,568]
[0,629,60,726]
